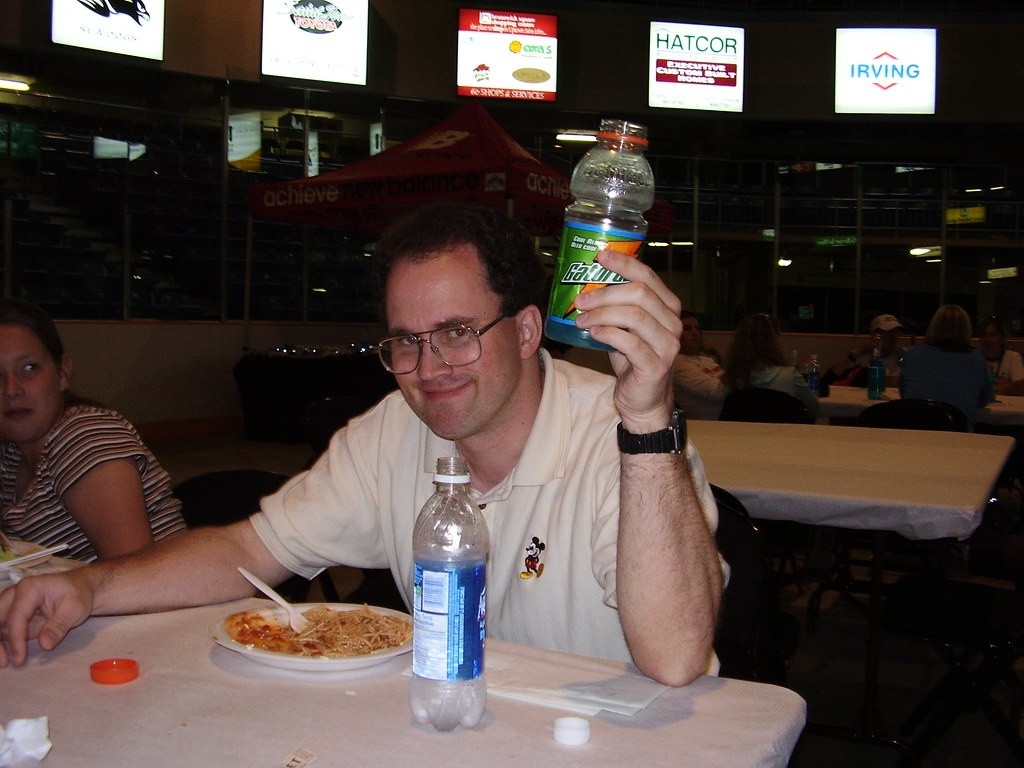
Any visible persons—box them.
[978,315,1024,396]
[823,314,910,387]
[670,310,726,421]
[0,299,189,564]
[716,312,821,418]
[0,200,731,685]
[898,304,992,432]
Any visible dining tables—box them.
[0,553,808,768]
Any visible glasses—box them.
[373,310,510,374]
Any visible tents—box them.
[242,100,675,351]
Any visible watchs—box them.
[616,409,687,455]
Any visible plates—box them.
[206,603,414,669]
[0,540,51,570]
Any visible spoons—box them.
[237,567,309,633]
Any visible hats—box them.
[870,314,903,331]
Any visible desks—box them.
[686,419,1017,752]
[815,386,1024,426]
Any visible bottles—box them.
[868,349,885,400]
[409,457,490,728]
[808,354,820,399]
[544,118,655,352]
[791,350,801,372]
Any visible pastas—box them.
[278,604,413,657]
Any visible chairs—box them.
[171,471,341,602]
[717,385,820,425]
[848,397,978,436]
[879,572,1024,768]
